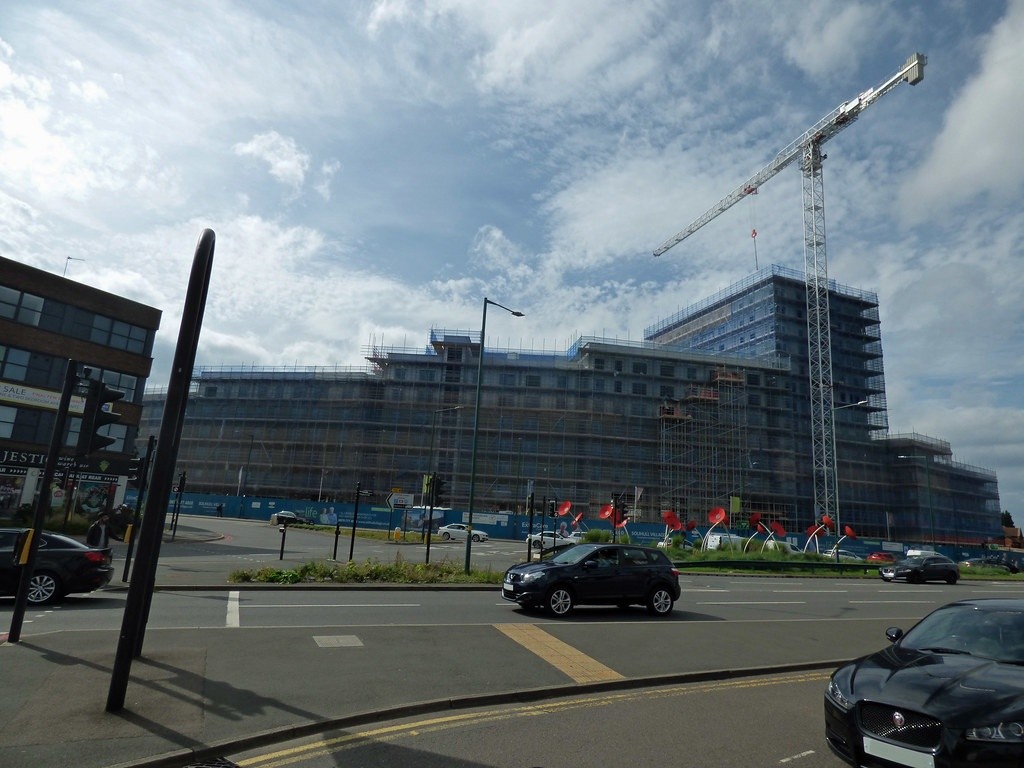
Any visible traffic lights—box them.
[620,501,628,522]
[127,456,149,491]
[77,379,125,451]
[55,466,71,489]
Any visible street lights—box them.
[422,405,464,542]
[463,295,525,577]
[235,429,254,518]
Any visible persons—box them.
[319,507,337,524]
[981,543,989,550]
[952,624,975,644]
[434,521,441,534]
[1005,624,1024,656]
[217,504,223,517]
[87,512,124,589]
[596,550,610,567]
[556,521,582,537]
[683,536,686,548]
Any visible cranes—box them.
[651,52,930,551]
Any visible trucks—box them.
[708,533,753,550]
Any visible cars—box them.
[503,543,682,618]
[527,530,578,549]
[962,558,1020,575]
[823,549,862,561]
[868,552,896,563]
[878,555,959,585]
[766,540,802,553]
[0,525,113,607]
[823,599,1024,768]
[656,537,697,551]
[436,522,491,544]
